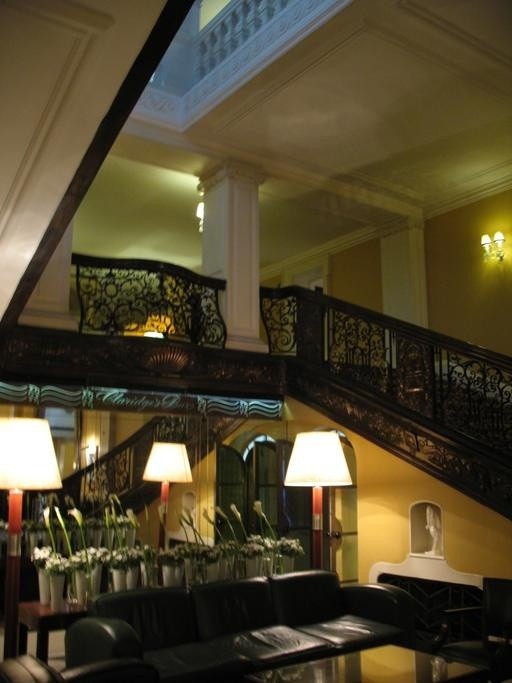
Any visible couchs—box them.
[64,568,435,683]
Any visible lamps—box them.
[0,417,63,659]
[480,230,505,262]
[143,440,195,587]
[283,429,354,569]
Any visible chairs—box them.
[436,575,511,683]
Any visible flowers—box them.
[28,494,304,577]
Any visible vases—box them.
[37,553,296,613]
